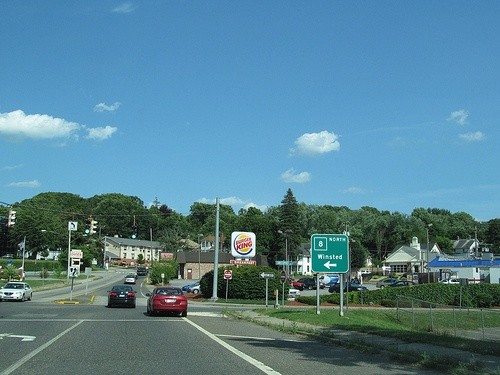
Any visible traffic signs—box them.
[310,233,350,274]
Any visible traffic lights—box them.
[8,210,17,227]
[131,226,137,239]
[85,220,91,234]
[88,217,98,235]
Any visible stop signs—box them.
[224,270,232,280]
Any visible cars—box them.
[329,282,369,294]
[182,281,201,294]
[124,266,147,284]
[0,281,32,302]
[293,278,325,291]
[376,277,415,291]
[318,274,339,286]
[439,278,470,285]
[145,286,188,317]
[107,285,137,308]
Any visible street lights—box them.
[197,233,204,281]
[277,229,288,273]
